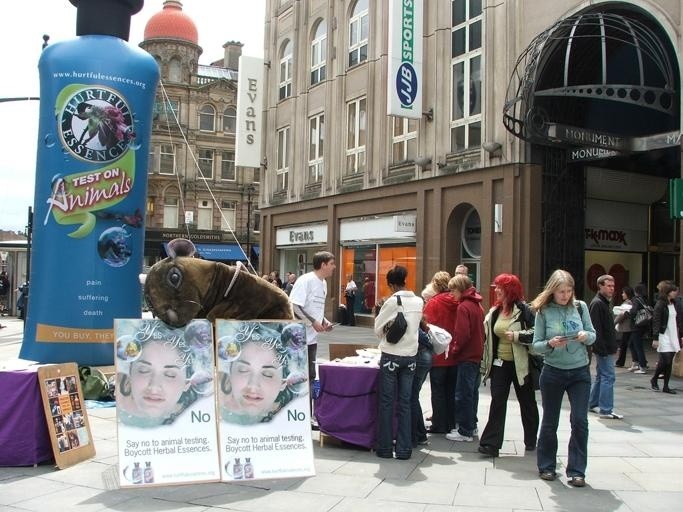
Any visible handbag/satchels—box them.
[634,297,654,326]
[672,350,683,377]
[426,323,452,355]
[530,356,543,390]
[386,295,407,343]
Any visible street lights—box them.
[237,182,256,275]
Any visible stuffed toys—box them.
[141,231,293,329]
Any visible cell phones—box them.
[560,333,578,341]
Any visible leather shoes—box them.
[525,445,535,450]
[479,445,499,457]
[651,381,676,394]
[541,473,554,479]
[572,477,585,486]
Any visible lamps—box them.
[413,155,433,169]
[481,141,505,159]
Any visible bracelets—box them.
[547,341,553,349]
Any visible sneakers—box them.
[589,407,624,419]
[628,365,646,373]
[419,426,478,445]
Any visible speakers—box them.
[495,204,502,233]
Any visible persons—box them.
[475,272,545,457]
[454,264,469,277]
[372,264,425,463]
[444,271,485,444]
[588,274,623,420]
[40,373,87,461]
[343,272,357,327]
[419,281,438,303]
[374,294,433,449]
[360,275,375,312]
[611,279,682,394]
[13,278,29,320]
[214,318,301,425]
[116,323,194,422]
[526,267,598,487]
[260,268,296,298]
[289,250,335,417]
[419,268,455,436]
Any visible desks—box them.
[1,359,53,467]
[319,347,399,452]
